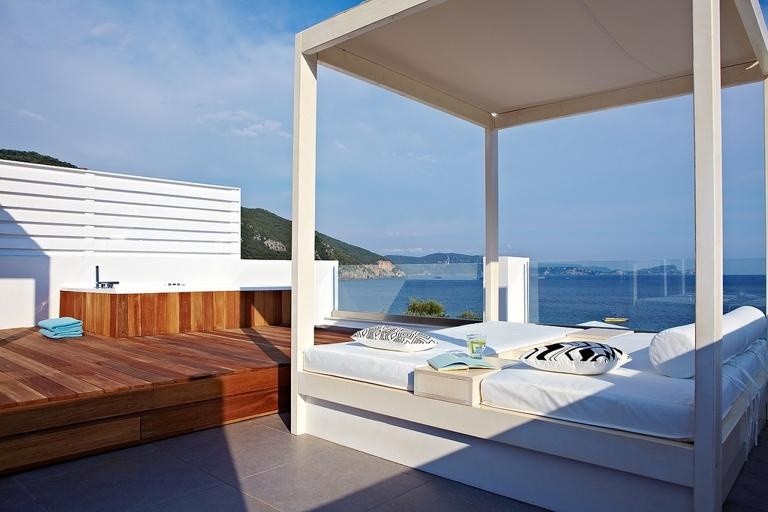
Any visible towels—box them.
[38,315,84,339]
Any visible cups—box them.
[465,333,486,360]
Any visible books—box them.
[426,349,497,372]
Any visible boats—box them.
[601,317,629,323]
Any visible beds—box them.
[288,0,768,510]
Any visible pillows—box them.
[648,305,768,380]
[518,339,633,375]
[349,324,438,351]
